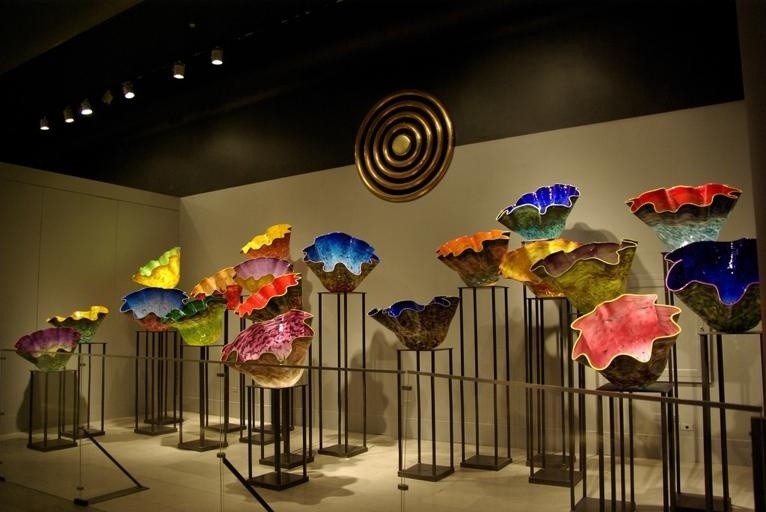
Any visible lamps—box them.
[38,41,225,132]
